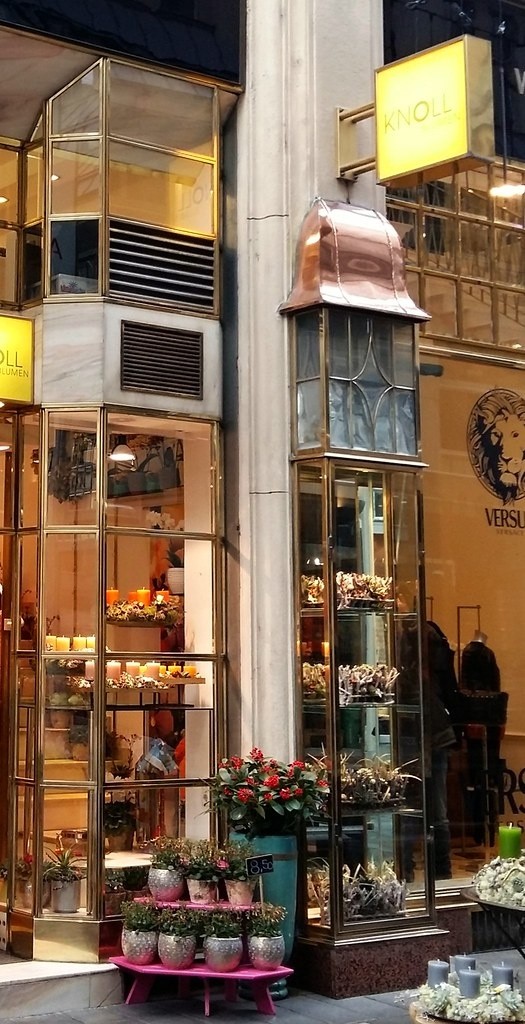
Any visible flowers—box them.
[140,835,260,889]
[198,747,330,829]
[146,511,184,567]
[15,835,87,886]
[108,436,183,476]
[108,731,142,775]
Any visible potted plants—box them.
[120,901,288,972]
[106,797,147,916]
[69,725,89,761]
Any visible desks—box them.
[461,887,525,959]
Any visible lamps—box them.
[110,433,135,461]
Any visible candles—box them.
[498,825,522,859]
[455,952,476,974]
[428,959,449,988]
[47,587,197,680]
[458,966,480,998]
[492,961,513,990]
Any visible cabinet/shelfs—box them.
[290,453,438,942]
[109,897,294,1016]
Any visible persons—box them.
[395,579,456,883]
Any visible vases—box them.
[149,867,255,906]
[50,710,73,728]
[108,462,183,498]
[19,879,81,913]
[230,830,298,1001]
[167,567,184,593]
